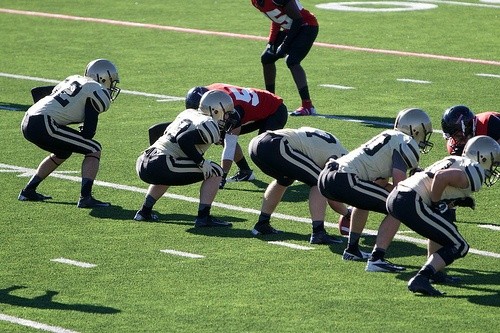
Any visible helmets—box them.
[394,108,434,154]
[84,59,121,101]
[199,89,234,132]
[441,105,477,154]
[462,135,500,187]
[185,87,209,110]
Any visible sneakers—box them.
[310,230,344,245]
[133,210,159,222]
[78,195,111,208]
[365,258,405,272]
[342,247,372,262]
[407,274,444,297]
[290,106,315,116]
[195,215,232,228]
[18,189,52,201]
[252,222,283,236]
[226,170,255,182]
[429,272,465,284]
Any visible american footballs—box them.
[339,205,353,236]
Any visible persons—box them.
[386,135,500,297]
[185,83,288,182]
[318,107,434,272]
[19,60,121,208]
[134,90,241,227]
[248,127,352,245]
[441,104,500,156]
[251,0,319,116]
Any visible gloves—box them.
[266,44,279,62]
[276,42,289,57]
[431,200,455,223]
[197,160,213,181]
[451,195,475,210]
[219,172,227,188]
[345,210,352,221]
